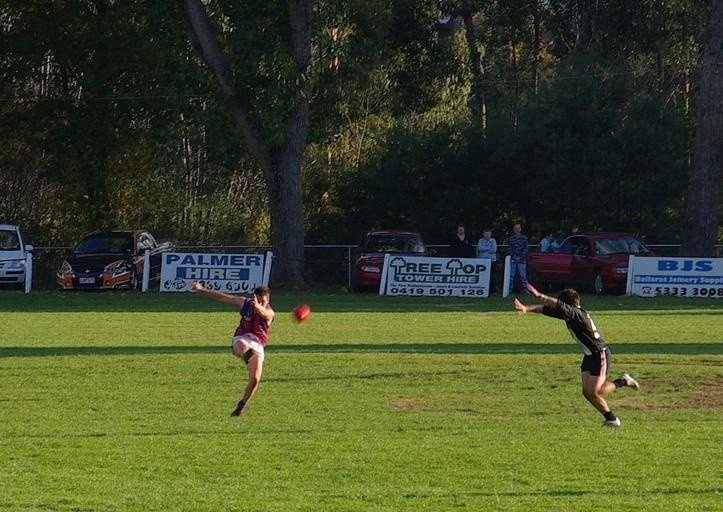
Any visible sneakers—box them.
[245,349,254,363]
[621,373,639,391]
[231,401,245,416]
[602,418,620,428]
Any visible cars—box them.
[0,224,34,285]
[342,229,429,292]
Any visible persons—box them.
[538,232,555,253]
[475,229,498,295]
[448,226,473,258]
[186,279,275,418]
[515,284,639,428]
[507,222,531,295]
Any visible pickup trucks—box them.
[55,230,174,291]
[512,232,656,296]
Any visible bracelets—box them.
[540,292,549,302]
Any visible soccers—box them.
[293,304,310,320]
